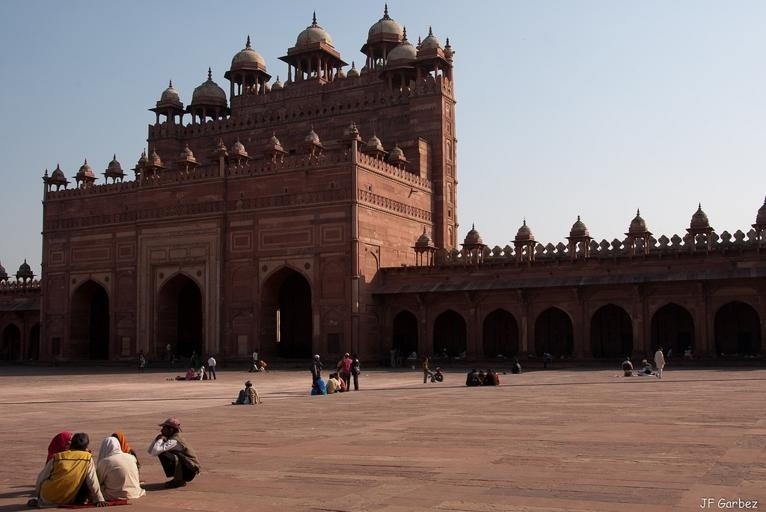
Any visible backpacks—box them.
[353,364,360,375]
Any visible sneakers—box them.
[163,479,187,489]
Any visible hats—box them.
[354,358,359,363]
[158,417,181,431]
[314,354,320,359]
[345,352,350,356]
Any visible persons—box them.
[232,381,263,405]
[112,433,145,483]
[622,357,633,376]
[638,359,652,374]
[466,369,500,386]
[148,418,201,488]
[310,353,360,395]
[654,347,666,378]
[166,343,216,380]
[512,360,521,374]
[137,352,146,373]
[45,432,94,463]
[248,350,268,372]
[422,357,436,383]
[27,434,109,508]
[88,437,146,502]
